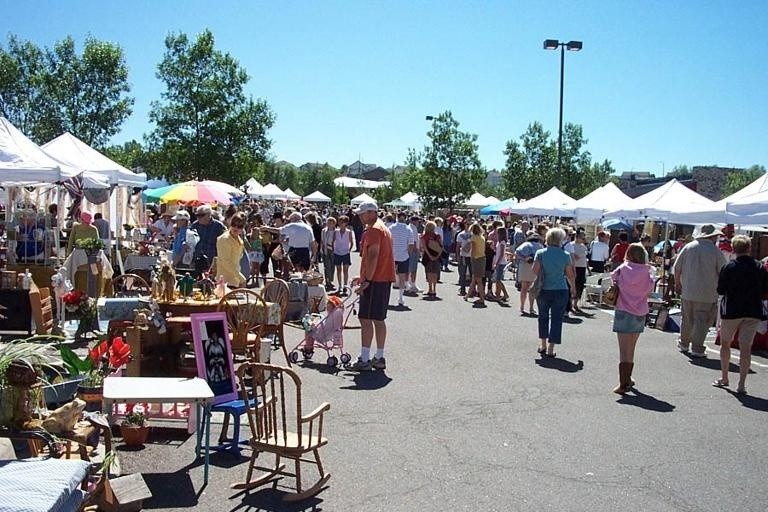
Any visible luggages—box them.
[285,278,309,321]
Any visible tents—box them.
[446,192,469,203]
[40,133,146,188]
[0,111,61,183]
[416,193,445,203]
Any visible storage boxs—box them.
[0,427,91,512]
[83,472,153,512]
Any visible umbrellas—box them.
[480,196,518,215]
[240,177,273,198]
[487,196,502,206]
[513,187,576,217]
[607,179,727,224]
[284,188,301,200]
[264,183,287,200]
[303,191,331,203]
[458,191,490,207]
[716,171,768,224]
[383,199,410,207]
[351,193,378,204]
[204,179,246,204]
[556,183,633,218]
[142,178,221,207]
[399,192,423,205]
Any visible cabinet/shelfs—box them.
[103,377,215,508]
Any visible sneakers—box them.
[325,281,337,292]
[690,351,709,358]
[346,357,372,371]
[485,291,510,302]
[370,357,386,369]
[459,288,485,308]
[441,267,450,272]
[406,285,423,294]
[398,298,405,305]
[678,339,690,352]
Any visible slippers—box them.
[712,379,729,387]
[735,387,748,396]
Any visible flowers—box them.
[60,337,134,387]
[122,411,145,425]
[60,289,85,304]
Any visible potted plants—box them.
[75,236,106,264]
[0,335,120,475]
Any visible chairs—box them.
[106,274,150,298]
[260,278,292,367]
[217,288,268,363]
[646,277,671,328]
[230,361,331,501]
[197,400,261,457]
[582,277,613,307]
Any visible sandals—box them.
[338,286,349,293]
[520,307,538,314]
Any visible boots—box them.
[627,362,635,387]
[615,362,628,395]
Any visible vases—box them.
[66,304,79,312]
[120,424,149,447]
[76,384,103,412]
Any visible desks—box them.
[98,295,282,325]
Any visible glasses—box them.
[232,224,244,229]
[581,236,587,241]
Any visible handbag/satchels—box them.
[587,241,594,259]
[604,267,619,306]
[530,249,545,298]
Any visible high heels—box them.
[428,292,437,298]
[538,346,547,355]
[546,352,557,360]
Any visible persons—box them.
[404,216,423,296]
[190,205,228,273]
[712,235,768,395]
[320,218,337,290]
[491,228,509,301]
[260,212,319,271]
[515,234,544,314]
[674,225,728,358]
[69,212,100,258]
[26,198,109,232]
[248,227,263,286]
[532,228,577,357]
[389,212,415,305]
[514,221,531,292]
[170,210,201,266]
[332,215,353,291]
[463,224,486,303]
[571,232,588,310]
[215,212,247,287]
[510,215,715,231]
[609,244,654,394]
[304,296,344,350]
[611,232,629,272]
[623,233,651,265]
[435,217,443,248]
[588,232,610,273]
[421,221,442,295]
[241,198,364,253]
[345,202,396,371]
[457,220,473,295]
[147,202,239,230]
[304,211,323,272]
[380,211,509,272]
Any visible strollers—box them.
[287,282,370,366]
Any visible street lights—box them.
[426,115,452,215]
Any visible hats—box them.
[144,209,156,216]
[170,211,191,221]
[528,233,542,242]
[695,225,725,240]
[285,213,303,221]
[159,211,175,219]
[195,205,211,216]
[328,295,342,307]
[352,201,377,215]
[81,212,92,225]
